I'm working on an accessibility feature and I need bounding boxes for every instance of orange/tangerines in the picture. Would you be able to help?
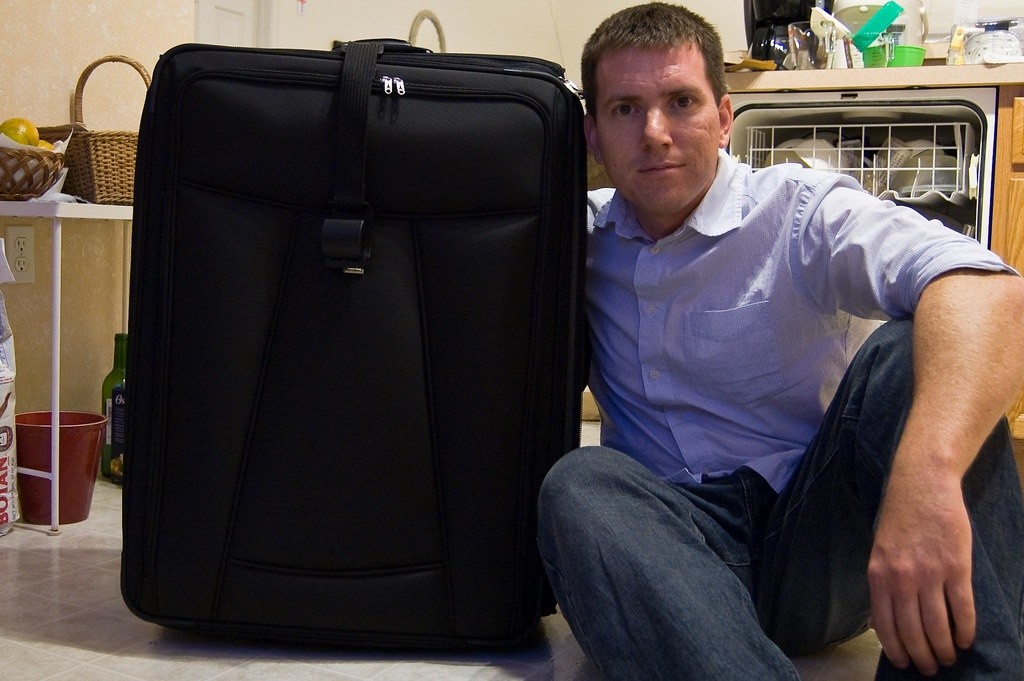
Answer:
[0,117,56,151]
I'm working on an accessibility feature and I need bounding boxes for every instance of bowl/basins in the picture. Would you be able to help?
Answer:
[764,132,958,198]
[862,43,927,68]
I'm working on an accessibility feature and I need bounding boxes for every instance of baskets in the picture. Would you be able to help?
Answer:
[43,54,152,206]
[0,147,66,202]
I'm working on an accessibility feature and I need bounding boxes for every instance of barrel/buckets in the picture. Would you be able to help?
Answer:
[15,410,109,527]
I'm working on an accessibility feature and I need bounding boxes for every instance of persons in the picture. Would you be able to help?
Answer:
[536,0,1023,681]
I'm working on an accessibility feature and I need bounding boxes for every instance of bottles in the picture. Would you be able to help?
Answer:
[100,331,127,479]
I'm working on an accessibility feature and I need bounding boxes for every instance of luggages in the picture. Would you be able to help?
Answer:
[119,30,591,655]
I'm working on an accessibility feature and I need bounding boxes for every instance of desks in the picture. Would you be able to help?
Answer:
[0,199,133,536]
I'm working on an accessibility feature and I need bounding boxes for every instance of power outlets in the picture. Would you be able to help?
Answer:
[6,226,34,283]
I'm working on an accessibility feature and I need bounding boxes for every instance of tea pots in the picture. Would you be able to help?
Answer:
[748,23,800,71]
